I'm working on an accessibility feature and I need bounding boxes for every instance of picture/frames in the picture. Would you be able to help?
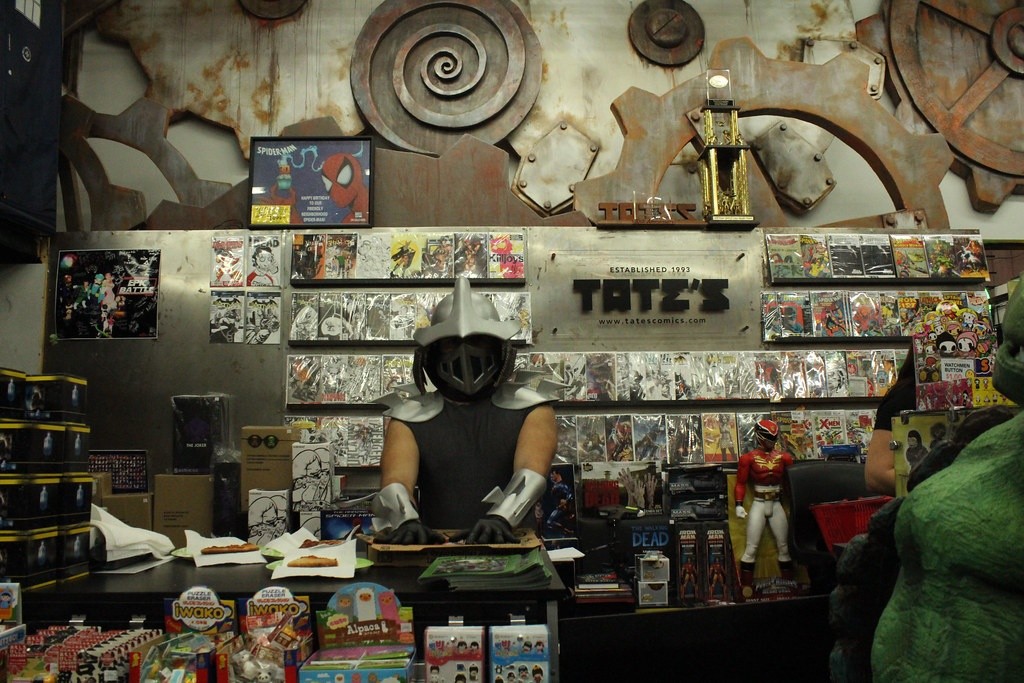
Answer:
[248,136,376,229]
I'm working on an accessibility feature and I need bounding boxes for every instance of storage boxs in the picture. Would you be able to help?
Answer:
[809,495,895,555]
[0,363,542,683]
[633,553,671,606]
[299,646,418,683]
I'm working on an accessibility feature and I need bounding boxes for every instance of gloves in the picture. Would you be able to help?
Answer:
[449,515,520,544]
[373,519,445,545]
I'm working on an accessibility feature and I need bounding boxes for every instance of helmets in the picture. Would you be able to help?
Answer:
[411,276,523,396]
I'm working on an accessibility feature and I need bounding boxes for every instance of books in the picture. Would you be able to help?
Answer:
[760,290,1020,412]
[54,249,162,341]
[285,347,909,593]
[208,289,533,344]
[765,234,991,282]
[209,231,527,287]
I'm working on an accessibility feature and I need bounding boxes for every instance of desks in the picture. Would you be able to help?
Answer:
[557,546,833,683]
[27,530,565,683]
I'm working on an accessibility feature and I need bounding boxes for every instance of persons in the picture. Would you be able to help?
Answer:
[710,558,728,596]
[864,343,916,492]
[380,292,558,544]
[682,557,697,597]
[734,420,794,580]
[62,273,116,335]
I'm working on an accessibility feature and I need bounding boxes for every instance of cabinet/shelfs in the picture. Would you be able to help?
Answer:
[0,0,65,265]
